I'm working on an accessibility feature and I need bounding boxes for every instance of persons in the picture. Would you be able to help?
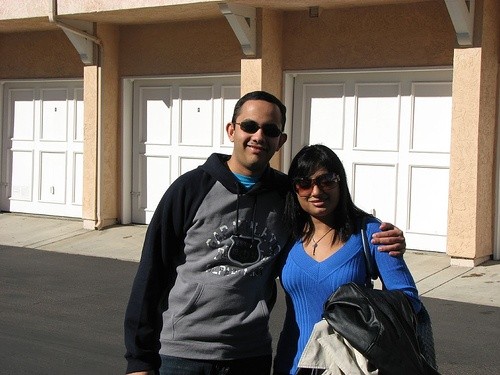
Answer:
[272,144,438,375]
[125,91,407,375]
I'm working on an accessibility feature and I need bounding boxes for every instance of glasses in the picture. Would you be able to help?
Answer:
[292,171,341,197]
[233,120,283,138]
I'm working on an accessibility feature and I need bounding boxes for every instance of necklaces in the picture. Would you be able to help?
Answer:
[311,228,333,255]
